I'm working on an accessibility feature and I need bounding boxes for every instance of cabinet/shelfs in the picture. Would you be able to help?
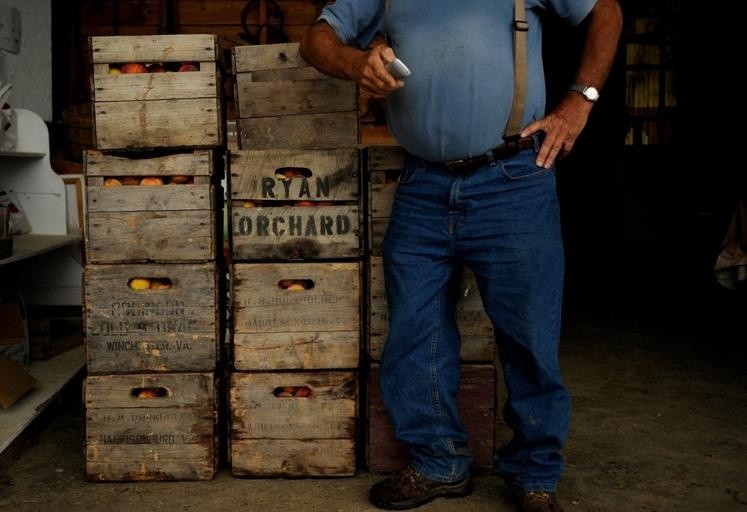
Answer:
[0,104,87,471]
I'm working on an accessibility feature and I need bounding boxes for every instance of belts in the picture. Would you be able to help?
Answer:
[409,129,546,176]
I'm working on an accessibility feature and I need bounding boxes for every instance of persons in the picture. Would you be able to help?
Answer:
[298,1,623,511]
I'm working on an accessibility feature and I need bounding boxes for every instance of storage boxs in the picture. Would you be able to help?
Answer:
[82,33,403,480]
[453,266,495,474]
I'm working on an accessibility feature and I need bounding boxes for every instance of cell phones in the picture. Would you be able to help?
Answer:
[385,58,411,80]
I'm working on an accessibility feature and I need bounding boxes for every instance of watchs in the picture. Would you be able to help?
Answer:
[571,84,601,104]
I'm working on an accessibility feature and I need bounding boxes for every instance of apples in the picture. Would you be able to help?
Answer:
[129,279,171,289]
[277,387,311,397]
[137,390,159,398]
[280,280,308,291]
[242,169,335,207]
[108,62,199,74]
[104,175,194,187]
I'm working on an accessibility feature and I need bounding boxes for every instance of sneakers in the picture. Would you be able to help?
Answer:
[367,463,475,511]
[502,482,564,512]
